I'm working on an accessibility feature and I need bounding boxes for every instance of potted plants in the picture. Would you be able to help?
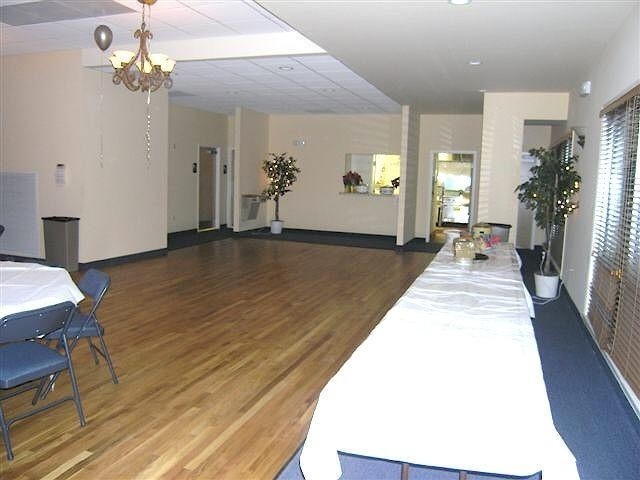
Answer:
[259,152,302,235]
[514,146,581,300]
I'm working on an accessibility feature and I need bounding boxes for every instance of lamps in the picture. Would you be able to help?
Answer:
[109,23,175,91]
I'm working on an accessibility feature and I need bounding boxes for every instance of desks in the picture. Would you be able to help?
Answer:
[0,259,71,318]
[315,238,580,480]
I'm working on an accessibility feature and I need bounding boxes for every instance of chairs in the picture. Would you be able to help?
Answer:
[40,268,119,400]
[1,301,85,461]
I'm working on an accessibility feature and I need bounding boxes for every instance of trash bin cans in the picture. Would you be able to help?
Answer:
[41,217,80,273]
[488,223,512,242]
